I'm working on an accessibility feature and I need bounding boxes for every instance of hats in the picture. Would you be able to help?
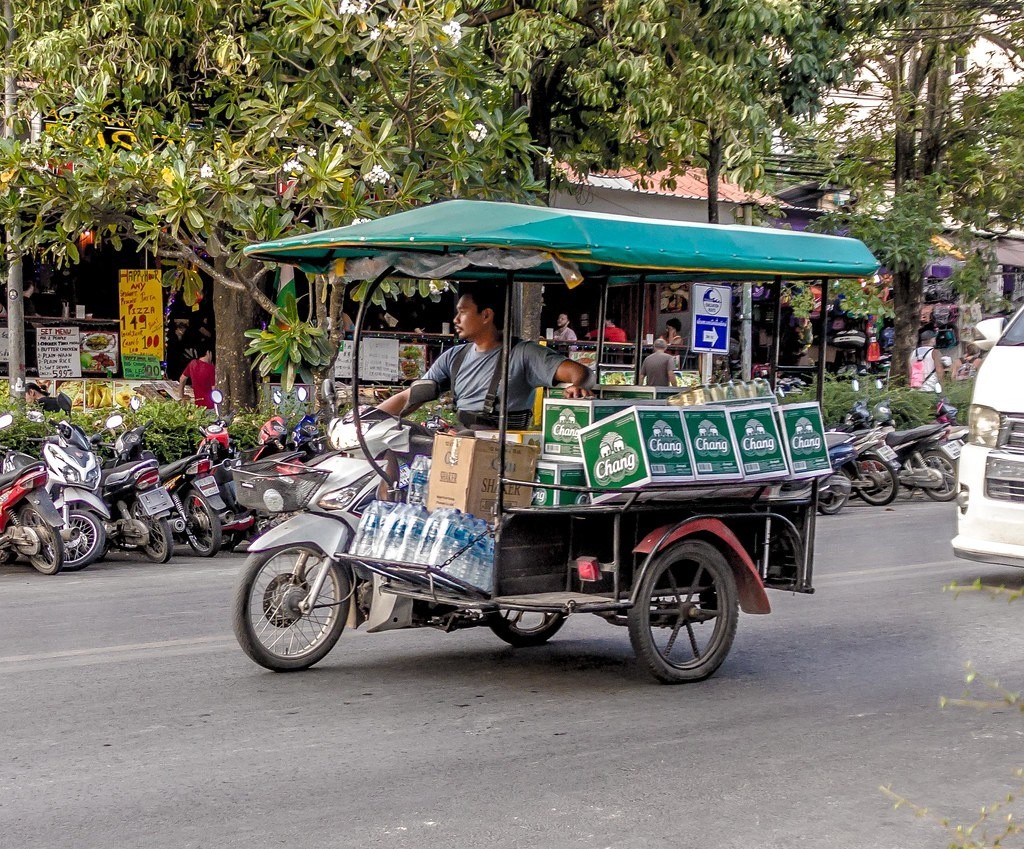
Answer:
[921,331,938,340]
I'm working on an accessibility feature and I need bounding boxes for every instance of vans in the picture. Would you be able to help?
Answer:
[950,303,1023,568]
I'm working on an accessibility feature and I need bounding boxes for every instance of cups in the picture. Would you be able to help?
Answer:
[63,307,69,317]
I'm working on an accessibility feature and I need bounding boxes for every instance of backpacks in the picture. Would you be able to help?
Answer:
[910,347,937,388]
[881,280,959,350]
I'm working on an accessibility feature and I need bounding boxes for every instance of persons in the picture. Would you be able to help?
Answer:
[638,337,678,387]
[50,246,88,317]
[167,296,216,412]
[553,311,578,341]
[908,330,948,393]
[366,303,426,332]
[23,281,45,329]
[951,343,986,382]
[584,312,627,343]
[373,284,597,440]
[25,382,68,416]
[658,317,685,345]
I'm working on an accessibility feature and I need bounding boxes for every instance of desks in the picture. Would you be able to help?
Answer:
[548,339,699,371]
[361,329,455,368]
[25,315,120,332]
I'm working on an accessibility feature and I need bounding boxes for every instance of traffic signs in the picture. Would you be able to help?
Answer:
[692,281,731,355]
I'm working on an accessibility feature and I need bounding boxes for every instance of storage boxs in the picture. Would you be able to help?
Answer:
[428,385,833,525]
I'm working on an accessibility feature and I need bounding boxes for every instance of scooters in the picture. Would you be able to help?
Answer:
[193,385,457,554]
[232,199,881,687]
[100,458,175,564]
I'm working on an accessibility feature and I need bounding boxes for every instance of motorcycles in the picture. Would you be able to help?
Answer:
[26,415,124,573]
[1,414,67,576]
[816,379,970,514]
[86,417,227,558]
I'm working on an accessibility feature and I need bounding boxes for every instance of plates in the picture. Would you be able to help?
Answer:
[82,333,116,353]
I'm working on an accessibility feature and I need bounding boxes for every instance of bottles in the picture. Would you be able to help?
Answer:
[348,498,495,594]
[406,454,433,508]
